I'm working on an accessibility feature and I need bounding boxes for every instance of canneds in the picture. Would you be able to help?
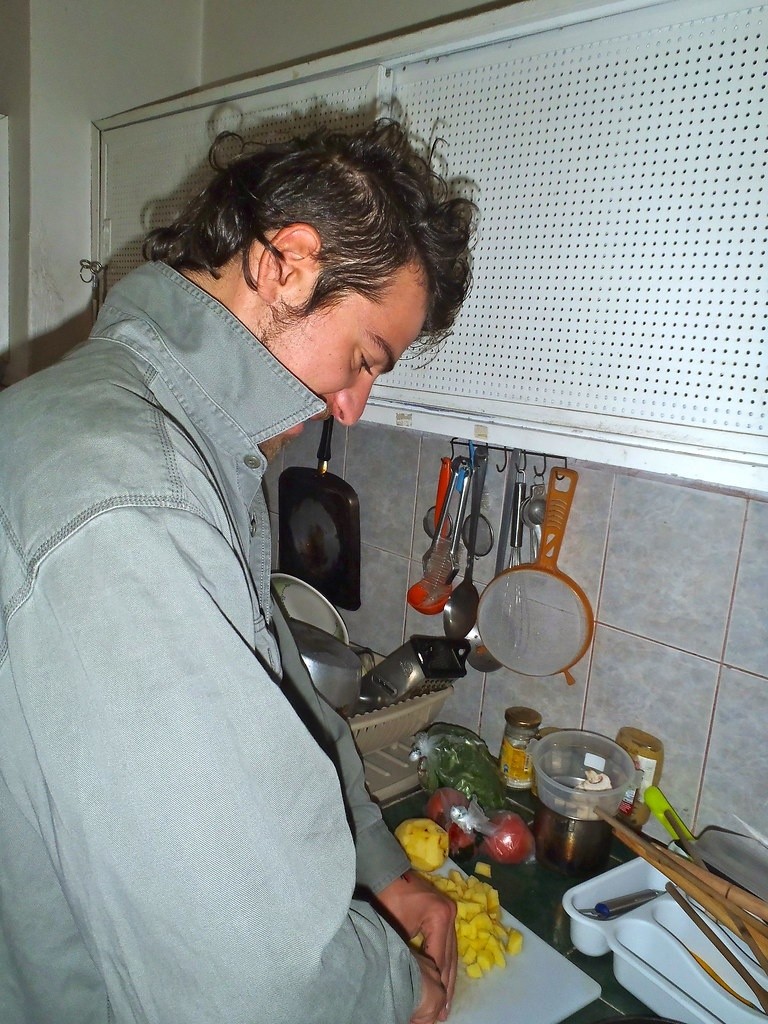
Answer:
[610,726,664,834]
[498,707,542,791]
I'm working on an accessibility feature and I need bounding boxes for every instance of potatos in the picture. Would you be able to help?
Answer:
[406,862,524,978]
[392,817,449,873]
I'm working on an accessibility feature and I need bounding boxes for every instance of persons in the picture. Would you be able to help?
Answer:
[1,110,492,1024]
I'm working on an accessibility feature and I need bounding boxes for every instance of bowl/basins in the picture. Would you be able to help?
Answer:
[526,732,636,820]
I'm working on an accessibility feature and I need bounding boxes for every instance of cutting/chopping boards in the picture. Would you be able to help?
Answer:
[426,859,602,1024]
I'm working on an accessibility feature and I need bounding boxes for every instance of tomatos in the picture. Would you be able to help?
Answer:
[476,815,536,864]
[428,788,474,847]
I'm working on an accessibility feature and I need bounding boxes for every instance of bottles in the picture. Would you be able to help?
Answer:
[616,728,664,835]
[497,707,543,791]
[530,725,563,799]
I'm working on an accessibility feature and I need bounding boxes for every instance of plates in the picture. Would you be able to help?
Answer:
[270,572,348,645]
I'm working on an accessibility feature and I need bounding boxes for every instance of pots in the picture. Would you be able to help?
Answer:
[277,416,362,611]
[476,465,594,686]
[531,800,613,876]
